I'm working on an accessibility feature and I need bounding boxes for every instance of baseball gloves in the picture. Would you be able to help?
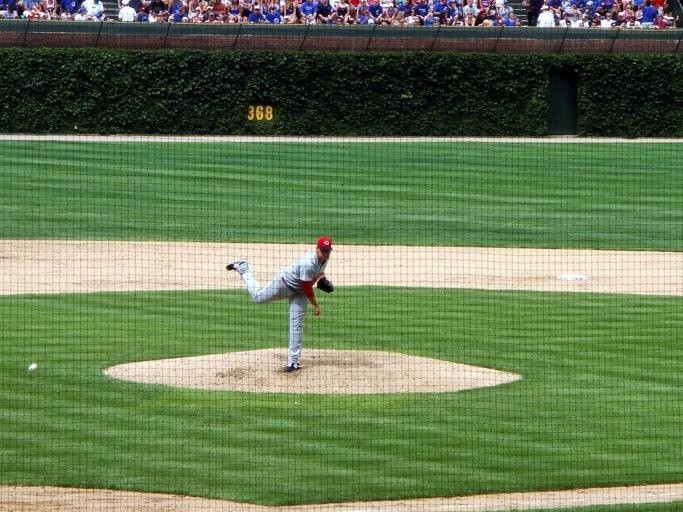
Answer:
[316,277,334,293]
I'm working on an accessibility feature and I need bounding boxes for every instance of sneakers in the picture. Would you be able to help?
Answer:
[288,362,302,371]
[225,257,248,271]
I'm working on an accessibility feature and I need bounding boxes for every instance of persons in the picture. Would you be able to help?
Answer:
[224,235,334,375]
[2,1,682,35]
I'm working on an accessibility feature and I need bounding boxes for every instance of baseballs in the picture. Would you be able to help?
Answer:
[28,363,39,373]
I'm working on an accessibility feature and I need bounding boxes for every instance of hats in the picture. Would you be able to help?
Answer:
[318,236,332,250]
